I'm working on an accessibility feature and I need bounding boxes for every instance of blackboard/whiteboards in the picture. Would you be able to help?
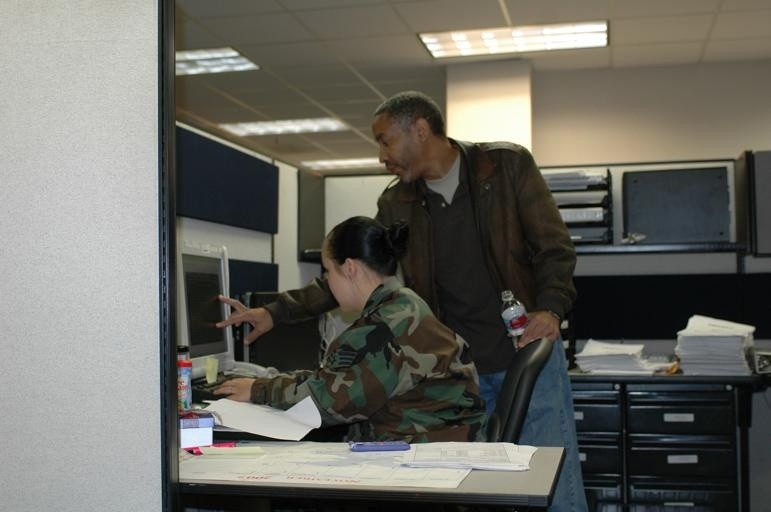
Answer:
[324,173,399,240]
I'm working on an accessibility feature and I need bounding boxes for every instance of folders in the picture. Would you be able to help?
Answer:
[747,345,770,374]
[542,170,614,245]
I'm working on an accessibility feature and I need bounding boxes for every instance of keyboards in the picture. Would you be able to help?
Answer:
[189,375,241,401]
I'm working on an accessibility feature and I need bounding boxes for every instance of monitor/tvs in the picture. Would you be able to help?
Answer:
[175,127,278,234]
[243,292,321,370]
[229,258,279,300]
[180,251,234,374]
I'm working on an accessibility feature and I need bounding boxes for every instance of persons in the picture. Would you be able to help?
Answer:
[212,216,487,442]
[216,92,589,511]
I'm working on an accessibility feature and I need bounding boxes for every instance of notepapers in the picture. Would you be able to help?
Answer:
[205,356,219,386]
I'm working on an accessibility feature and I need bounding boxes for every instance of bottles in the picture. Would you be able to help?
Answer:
[500,290,534,354]
[176,345,193,416]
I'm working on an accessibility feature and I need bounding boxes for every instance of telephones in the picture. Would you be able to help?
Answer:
[221,359,279,380]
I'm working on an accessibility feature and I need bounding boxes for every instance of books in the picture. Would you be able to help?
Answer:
[542,169,611,244]
[573,337,655,376]
[675,314,755,376]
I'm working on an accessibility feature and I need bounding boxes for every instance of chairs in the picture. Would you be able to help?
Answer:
[487,336,552,444]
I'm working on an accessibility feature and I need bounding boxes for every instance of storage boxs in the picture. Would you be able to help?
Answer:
[584,480,626,511]
[575,394,624,434]
[575,438,624,480]
[629,441,739,482]
[626,396,736,438]
[629,485,740,512]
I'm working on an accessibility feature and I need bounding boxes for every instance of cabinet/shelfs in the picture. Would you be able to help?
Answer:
[296,153,771,275]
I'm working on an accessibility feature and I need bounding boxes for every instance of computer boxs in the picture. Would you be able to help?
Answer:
[620,166,731,246]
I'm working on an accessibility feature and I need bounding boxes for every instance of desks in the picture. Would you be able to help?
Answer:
[565,336,770,511]
[180,433,567,511]
